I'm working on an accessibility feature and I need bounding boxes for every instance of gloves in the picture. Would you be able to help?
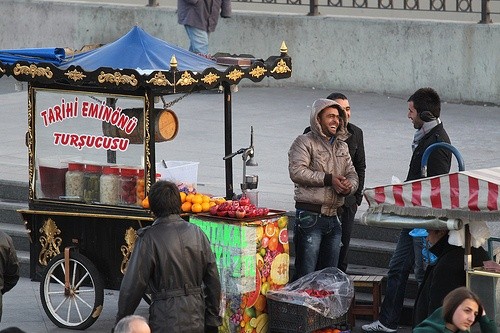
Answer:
[204,325,219,333]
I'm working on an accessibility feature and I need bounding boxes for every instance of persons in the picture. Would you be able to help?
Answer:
[361,87,453,333]
[412,286,500,333]
[177,0,233,56]
[0,229,20,325]
[114,315,152,333]
[288,100,360,283]
[108,180,221,333]
[412,215,495,333]
[301,93,365,274]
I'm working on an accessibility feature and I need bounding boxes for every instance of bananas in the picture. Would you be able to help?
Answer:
[254,313,269,333]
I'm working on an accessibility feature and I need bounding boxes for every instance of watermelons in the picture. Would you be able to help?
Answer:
[246,269,261,309]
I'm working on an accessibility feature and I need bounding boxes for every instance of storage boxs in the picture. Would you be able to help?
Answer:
[154,160,200,191]
[265,285,350,333]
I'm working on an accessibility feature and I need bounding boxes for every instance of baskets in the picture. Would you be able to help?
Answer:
[267,291,355,333]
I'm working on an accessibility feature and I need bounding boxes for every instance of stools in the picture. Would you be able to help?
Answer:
[349,276,384,326]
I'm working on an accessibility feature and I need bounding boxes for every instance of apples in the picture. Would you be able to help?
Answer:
[119,175,138,203]
[209,197,269,218]
[297,288,333,299]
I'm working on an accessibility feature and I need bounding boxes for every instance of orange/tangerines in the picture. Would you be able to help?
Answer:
[312,328,342,333]
[236,217,290,333]
[141,192,225,213]
[136,177,145,206]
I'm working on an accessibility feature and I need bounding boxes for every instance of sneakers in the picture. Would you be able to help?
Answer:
[361,320,398,333]
